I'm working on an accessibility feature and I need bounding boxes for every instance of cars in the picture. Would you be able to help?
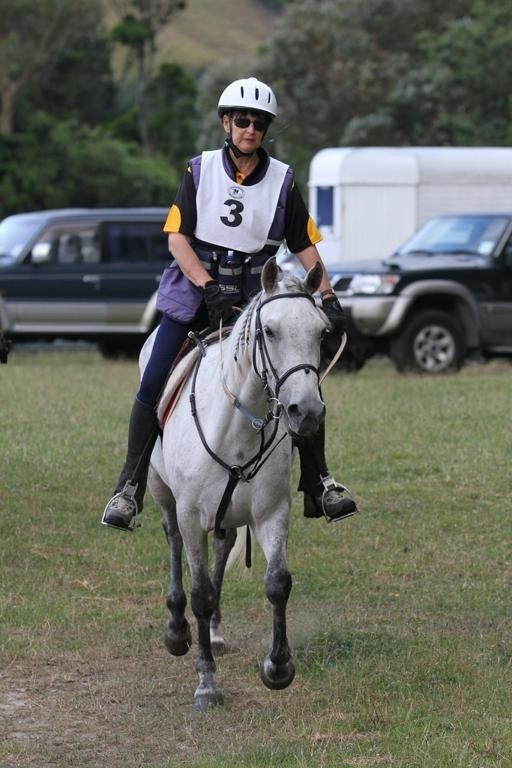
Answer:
[293,213,512,373]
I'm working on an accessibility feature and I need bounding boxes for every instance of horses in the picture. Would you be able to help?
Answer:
[138,256,328,713]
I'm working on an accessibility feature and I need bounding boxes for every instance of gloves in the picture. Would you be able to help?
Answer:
[320,298,347,333]
[195,279,234,322]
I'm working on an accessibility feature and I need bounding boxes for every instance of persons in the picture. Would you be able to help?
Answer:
[105,77,357,529]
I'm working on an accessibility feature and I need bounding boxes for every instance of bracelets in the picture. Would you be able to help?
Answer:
[320,289,334,301]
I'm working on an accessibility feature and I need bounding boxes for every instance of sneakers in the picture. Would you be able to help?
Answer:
[104,493,135,528]
[303,487,356,519]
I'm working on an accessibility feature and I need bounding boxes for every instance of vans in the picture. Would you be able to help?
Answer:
[1,207,173,363]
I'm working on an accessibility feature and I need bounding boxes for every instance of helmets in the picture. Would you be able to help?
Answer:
[216,76,278,118]
